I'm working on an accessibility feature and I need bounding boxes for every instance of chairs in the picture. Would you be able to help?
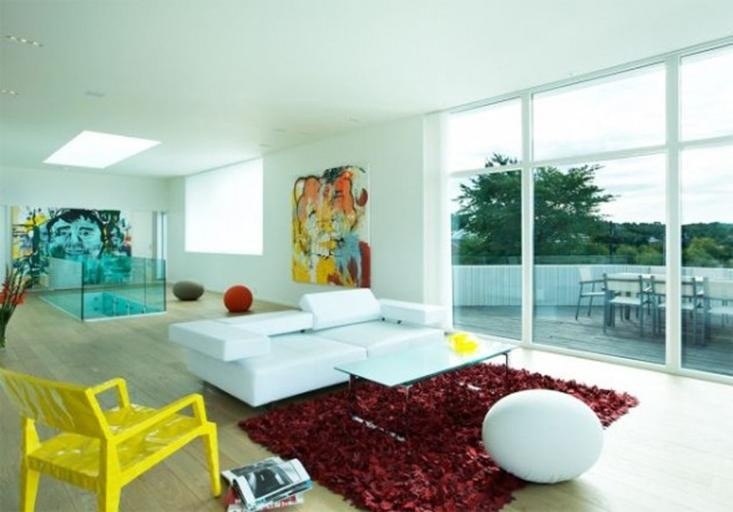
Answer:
[0,367,223,512]
[573,270,711,348]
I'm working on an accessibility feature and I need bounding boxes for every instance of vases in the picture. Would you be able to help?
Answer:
[0,250,40,319]
[1,304,17,348]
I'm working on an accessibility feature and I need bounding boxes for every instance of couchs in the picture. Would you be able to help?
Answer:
[167,298,452,408]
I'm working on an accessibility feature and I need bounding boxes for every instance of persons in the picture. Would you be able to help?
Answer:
[46,210,106,262]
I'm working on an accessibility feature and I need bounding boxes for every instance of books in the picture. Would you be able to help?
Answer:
[220,455,313,512]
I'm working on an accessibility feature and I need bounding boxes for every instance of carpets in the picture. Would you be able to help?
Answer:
[237,361,638,512]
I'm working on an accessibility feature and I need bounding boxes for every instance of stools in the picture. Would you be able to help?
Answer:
[481,389,608,486]
[172,281,204,302]
[224,285,252,312]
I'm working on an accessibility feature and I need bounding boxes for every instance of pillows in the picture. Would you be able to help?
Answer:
[299,287,384,331]
